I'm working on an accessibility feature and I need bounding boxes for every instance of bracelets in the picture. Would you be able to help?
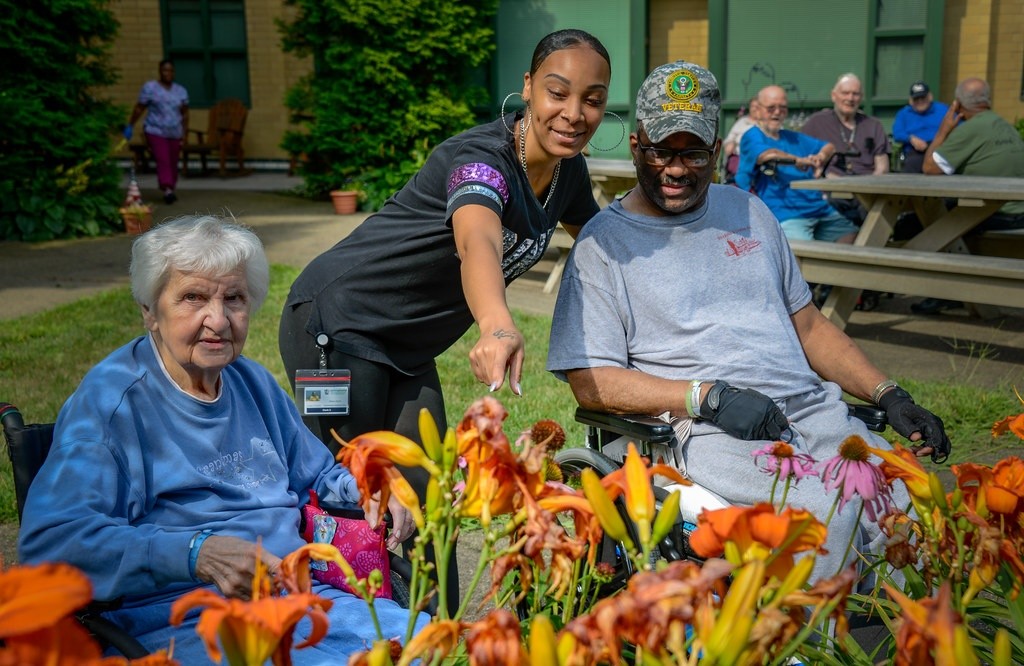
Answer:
[685,379,702,419]
[189,532,213,579]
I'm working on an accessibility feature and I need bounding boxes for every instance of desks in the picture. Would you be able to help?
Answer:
[788,175,1024,351]
[540,157,638,294]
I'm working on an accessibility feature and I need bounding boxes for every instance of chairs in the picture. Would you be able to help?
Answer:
[182,97,248,176]
[128,104,192,172]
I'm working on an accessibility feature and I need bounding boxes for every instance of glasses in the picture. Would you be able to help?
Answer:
[637,134,718,168]
[760,103,788,113]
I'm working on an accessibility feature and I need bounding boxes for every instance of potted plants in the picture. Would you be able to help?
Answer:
[118,204,155,234]
[329,181,360,213]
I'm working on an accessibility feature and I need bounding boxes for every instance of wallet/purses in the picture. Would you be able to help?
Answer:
[303,504,392,600]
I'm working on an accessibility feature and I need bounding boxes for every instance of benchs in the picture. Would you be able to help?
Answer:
[788,238,1024,310]
[547,228,576,250]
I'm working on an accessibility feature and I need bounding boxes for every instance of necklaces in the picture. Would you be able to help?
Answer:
[520,119,560,209]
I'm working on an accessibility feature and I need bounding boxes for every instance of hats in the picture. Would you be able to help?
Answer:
[910,82,928,100]
[635,60,721,147]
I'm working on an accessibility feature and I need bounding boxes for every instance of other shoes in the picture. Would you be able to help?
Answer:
[164,193,177,205]
[818,289,880,312]
[911,298,965,313]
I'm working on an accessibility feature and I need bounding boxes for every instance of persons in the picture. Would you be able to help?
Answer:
[310,391,320,401]
[544,59,952,666]
[277,28,612,620]
[17,206,435,666]
[130,59,189,204]
[724,71,1024,316]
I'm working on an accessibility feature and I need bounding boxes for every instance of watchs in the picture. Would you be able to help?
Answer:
[872,379,898,405]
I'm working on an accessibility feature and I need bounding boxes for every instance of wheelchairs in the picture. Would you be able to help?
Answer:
[0,399,448,666]
[510,401,895,666]
[725,147,880,313]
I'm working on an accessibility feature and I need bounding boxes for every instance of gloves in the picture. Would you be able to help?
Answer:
[700,380,789,442]
[124,125,133,141]
